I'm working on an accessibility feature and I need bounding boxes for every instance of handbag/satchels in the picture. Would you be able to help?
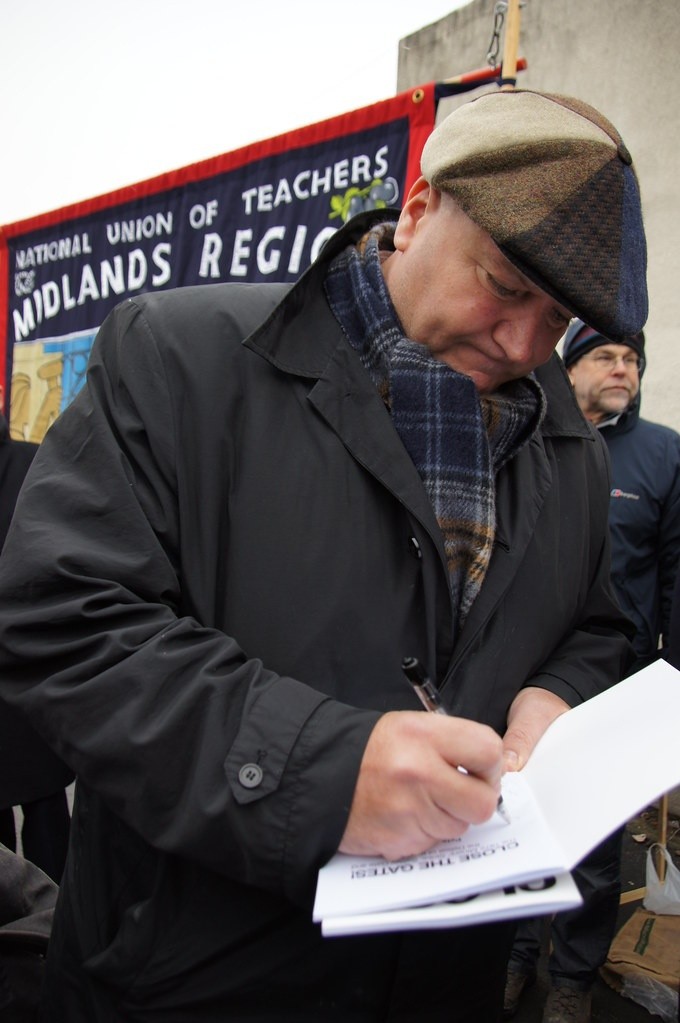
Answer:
[643,843,680,915]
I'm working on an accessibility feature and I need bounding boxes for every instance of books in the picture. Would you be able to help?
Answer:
[313,658,680,937]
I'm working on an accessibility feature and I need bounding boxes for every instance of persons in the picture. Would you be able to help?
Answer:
[0,414,77,885]
[504,321,680,1023]
[0,90,650,1023]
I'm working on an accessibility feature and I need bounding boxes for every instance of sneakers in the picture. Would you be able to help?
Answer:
[503,967,537,1018]
[542,986,591,1023]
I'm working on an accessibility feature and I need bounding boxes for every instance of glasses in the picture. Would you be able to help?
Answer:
[581,354,644,371]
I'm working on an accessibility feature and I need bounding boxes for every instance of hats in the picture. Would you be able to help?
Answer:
[562,318,646,379]
[420,89,649,344]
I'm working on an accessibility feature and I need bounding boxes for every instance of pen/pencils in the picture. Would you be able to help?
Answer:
[403,657,512,826]
